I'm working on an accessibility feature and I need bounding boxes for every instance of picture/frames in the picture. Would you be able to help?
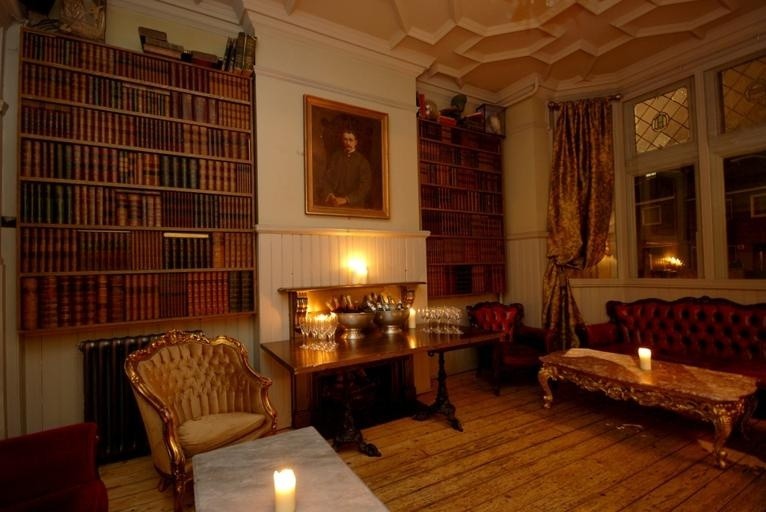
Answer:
[303,94,391,219]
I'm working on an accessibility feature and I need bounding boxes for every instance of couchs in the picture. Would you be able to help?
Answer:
[124,330,278,512]
[467,303,556,395]
[576,296,766,390]
[0,422,109,512]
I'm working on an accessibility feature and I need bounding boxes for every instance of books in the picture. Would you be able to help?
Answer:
[23,31,250,102]
[420,139,500,172]
[21,138,252,193]
[21,271,254,331]
[138,25,256,76]
[419,120,501,153]
[20,183,252,229]
[419,162,501,193]
[20,226,253,272]
[416,90,483,131]
[427,237,504,264]
[22,100,252,162]
[428,264,505,296]
[421,185,502,214]
[422,210,503,238]
[23,62,250,131]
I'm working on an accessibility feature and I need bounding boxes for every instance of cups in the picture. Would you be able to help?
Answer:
[638,347,652,370]
[418,307,460,335]
[298,312,339,353]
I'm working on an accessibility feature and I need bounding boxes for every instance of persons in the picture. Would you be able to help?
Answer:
[322,129,372,208]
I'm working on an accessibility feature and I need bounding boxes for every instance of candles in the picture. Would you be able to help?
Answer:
[639,346,651,370]
[347,258,370,286]
[408,307,417,328]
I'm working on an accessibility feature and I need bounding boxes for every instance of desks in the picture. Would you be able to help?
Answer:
[260,322,506,457]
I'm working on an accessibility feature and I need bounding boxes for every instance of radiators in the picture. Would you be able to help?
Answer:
[78,333,207,463]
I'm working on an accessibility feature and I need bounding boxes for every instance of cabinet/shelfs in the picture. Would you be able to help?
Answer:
[22,32,254,330]
[418,118,508,299]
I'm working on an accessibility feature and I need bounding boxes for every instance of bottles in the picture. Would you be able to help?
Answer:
[341,294,348,313]
[332,297,341,309]
[325,302,335,313]
[361,291,403,312]
[346,295,355,313]
